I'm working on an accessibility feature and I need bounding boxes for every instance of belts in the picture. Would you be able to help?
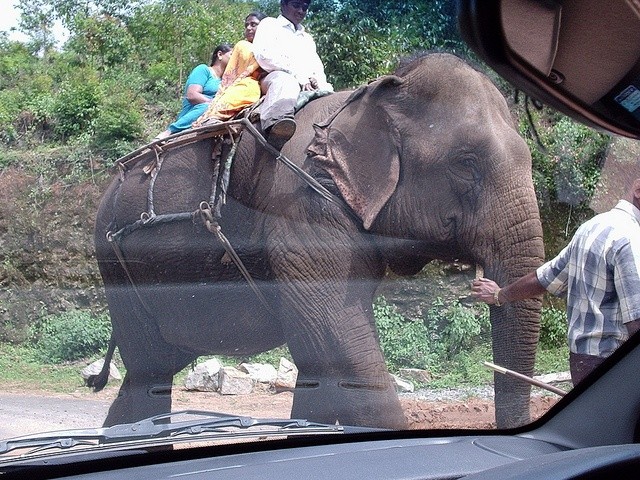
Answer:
[568,350,608,363]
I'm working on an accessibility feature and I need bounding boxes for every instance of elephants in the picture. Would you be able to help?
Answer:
[87,51,546,451]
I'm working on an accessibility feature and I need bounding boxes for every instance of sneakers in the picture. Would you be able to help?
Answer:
[266,117,298,153]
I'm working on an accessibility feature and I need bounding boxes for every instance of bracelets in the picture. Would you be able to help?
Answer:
[494,289,502,307]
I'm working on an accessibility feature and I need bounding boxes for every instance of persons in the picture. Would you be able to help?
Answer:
[153,44,234,144]
[252,0,332,151]
[191,13,268,127]
[467,176,639,386]
